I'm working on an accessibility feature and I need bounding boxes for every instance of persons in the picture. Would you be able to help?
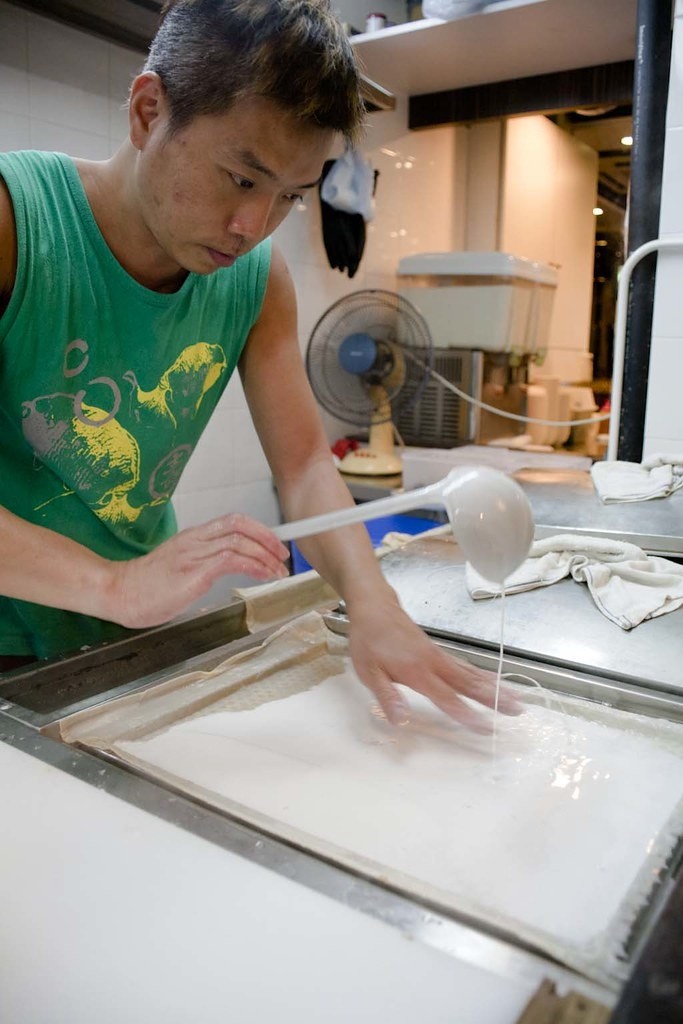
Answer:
[0,0,528,738]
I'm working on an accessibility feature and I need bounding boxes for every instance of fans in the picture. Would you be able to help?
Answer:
[306,285,434,476]
